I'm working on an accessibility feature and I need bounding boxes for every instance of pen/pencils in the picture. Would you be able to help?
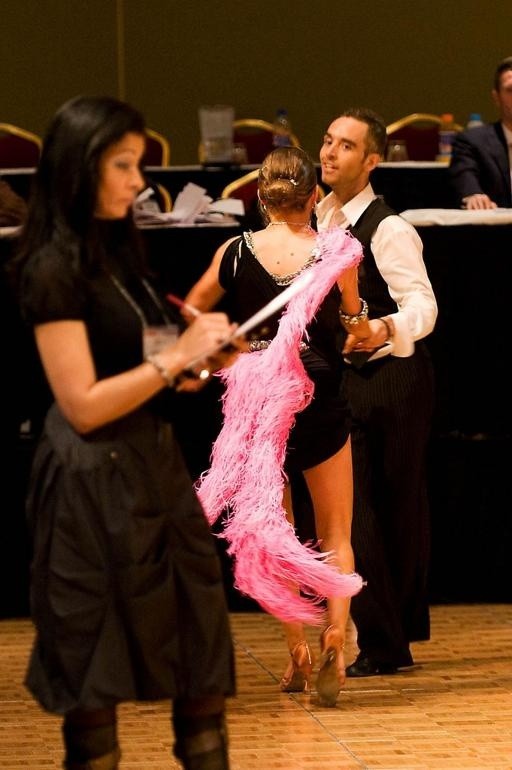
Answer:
[167,295,200,318]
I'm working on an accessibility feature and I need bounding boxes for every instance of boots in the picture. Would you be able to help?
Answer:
[62,720,119,770]
[173,712,229,770]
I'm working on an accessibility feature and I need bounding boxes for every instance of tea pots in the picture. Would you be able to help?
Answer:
[197,103,236,171]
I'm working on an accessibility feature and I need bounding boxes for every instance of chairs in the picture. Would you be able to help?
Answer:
[0,121,45,167]
[371,113,464,162]
[134,126,172,167]
[196,117,302,163]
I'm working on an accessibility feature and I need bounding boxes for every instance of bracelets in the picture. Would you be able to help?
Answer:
[377,318,391,341]
[338,298,369,325]
[145,354,176,389]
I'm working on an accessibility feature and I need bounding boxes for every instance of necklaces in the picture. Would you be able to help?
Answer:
[265,222,306,229]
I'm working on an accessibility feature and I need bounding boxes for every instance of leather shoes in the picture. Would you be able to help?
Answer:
[345,649,414,677]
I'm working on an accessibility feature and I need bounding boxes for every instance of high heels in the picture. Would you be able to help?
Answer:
[317,624,345,699]
[279,640,314,693]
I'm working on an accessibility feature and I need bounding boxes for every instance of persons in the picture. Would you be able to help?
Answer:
[178,146,373,708]
[308,104,439,678]
[449,55,512,210]
[1,92,241,770]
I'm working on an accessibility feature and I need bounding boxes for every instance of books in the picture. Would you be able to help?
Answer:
[133,182,245,227]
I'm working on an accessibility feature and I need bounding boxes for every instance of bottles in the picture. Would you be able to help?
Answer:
[271,107,292,147]
[466,112,485,130]
[438,112,458,165]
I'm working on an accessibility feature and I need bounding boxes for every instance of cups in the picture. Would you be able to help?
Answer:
[230,143,247,165]
[385,139,409,163]
